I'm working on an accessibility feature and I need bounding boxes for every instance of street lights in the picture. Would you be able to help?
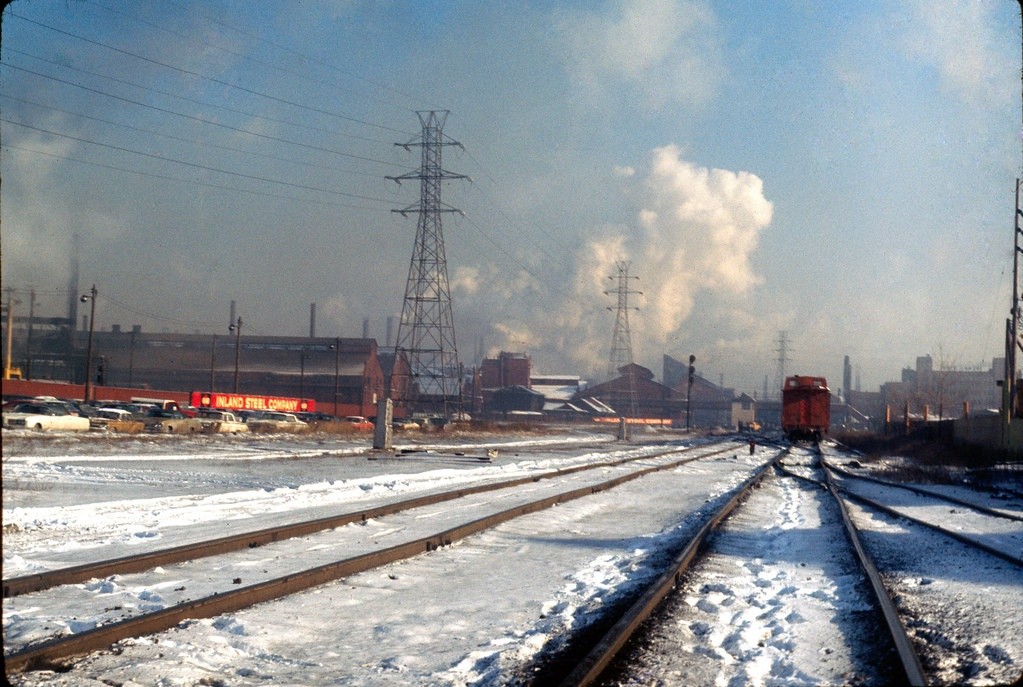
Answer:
[228,315,242,394]
[80,283,97,404]
[330,337,340,416]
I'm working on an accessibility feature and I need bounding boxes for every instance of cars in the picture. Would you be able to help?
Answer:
[2,395,375,434]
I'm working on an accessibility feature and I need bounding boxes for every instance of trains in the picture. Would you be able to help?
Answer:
[781,375,831,444]
